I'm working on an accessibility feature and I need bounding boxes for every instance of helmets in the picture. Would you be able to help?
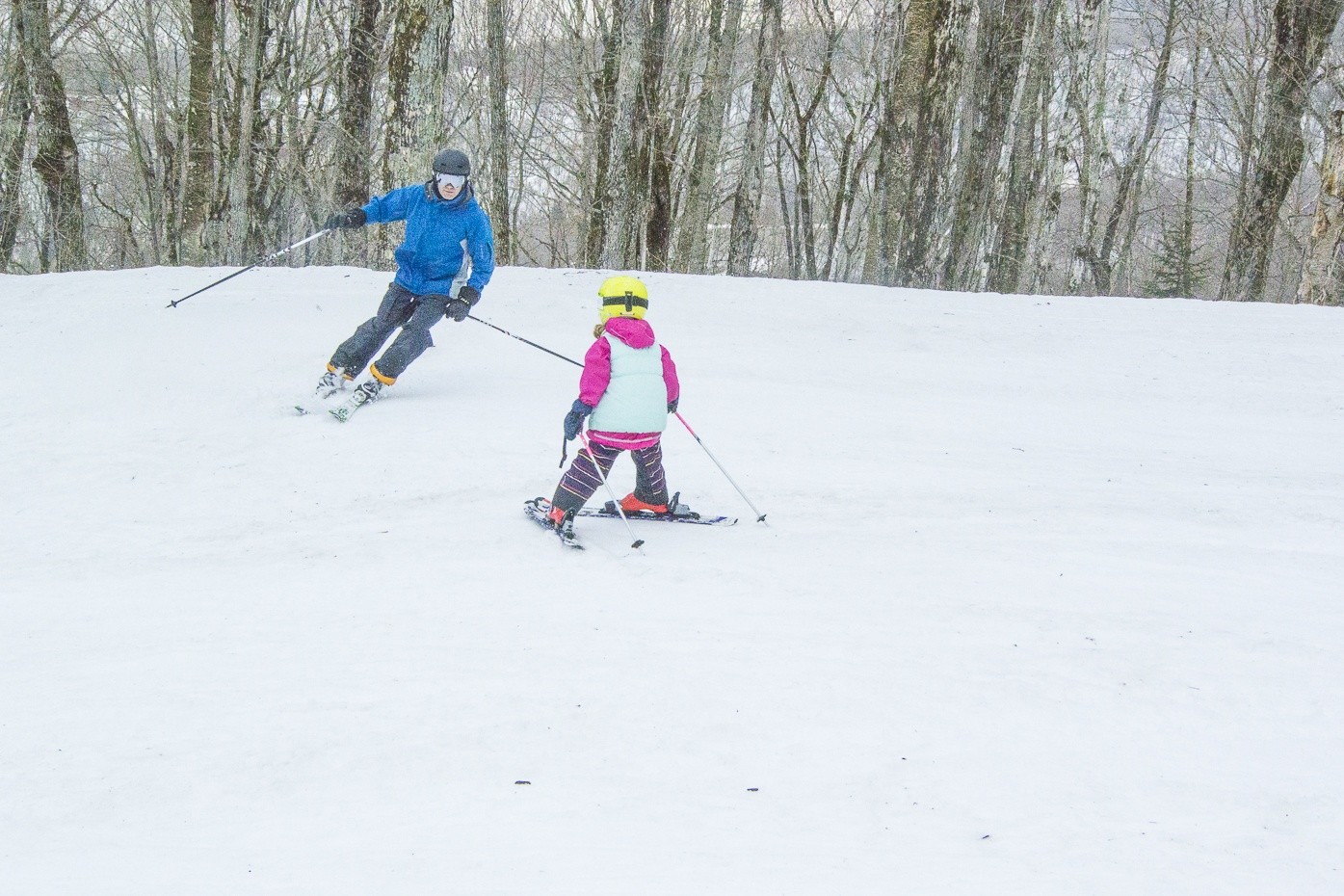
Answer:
[432,147,470,177]
[596,276,649,324]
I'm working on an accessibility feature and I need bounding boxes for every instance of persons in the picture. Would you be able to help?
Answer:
[546,275,682,529]
[318,146,497,404]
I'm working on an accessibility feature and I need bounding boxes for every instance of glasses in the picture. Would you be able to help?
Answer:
[435,172,466,188]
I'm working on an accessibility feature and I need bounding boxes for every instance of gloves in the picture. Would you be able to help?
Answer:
[563,399,592,442]
[442,285,478,322]
[323,208,368,231]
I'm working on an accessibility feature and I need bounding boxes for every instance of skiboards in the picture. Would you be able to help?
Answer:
[521,496,766,554]
[293,368,375,422]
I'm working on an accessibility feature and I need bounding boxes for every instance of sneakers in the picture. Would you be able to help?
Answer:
[316,370,346,392]
[352,377,383,404]
[545,503,566,526]
[618,493,668,515]
